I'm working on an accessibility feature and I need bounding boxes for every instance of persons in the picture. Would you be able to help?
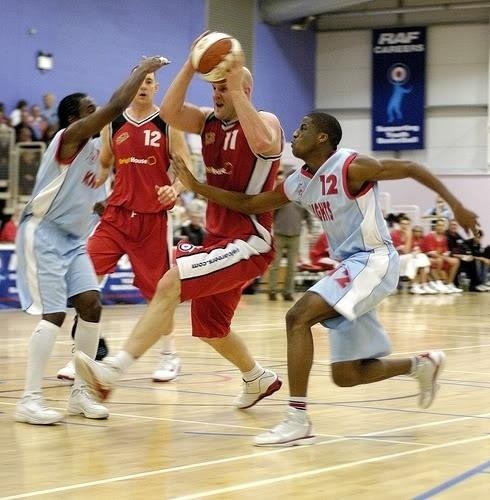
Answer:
[169,112,483,446]
[57,64,193,384]
[68,28,286,410]
[10,54,172,426]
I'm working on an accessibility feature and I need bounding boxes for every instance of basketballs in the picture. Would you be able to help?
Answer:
[191,32,241,83]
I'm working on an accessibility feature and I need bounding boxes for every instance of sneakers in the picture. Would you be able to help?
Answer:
[67,383,109,419]
[237,368,281,411]
[15,393,62,424]
[253,406,315,447]
[413,349,445,410]
[74,351,118,402]
[284,293,294,301]
[269,291,277,301]
[152,353,181,382]
[56,343,75,383]
[70,317,106,359]
[409,280,490,294]
